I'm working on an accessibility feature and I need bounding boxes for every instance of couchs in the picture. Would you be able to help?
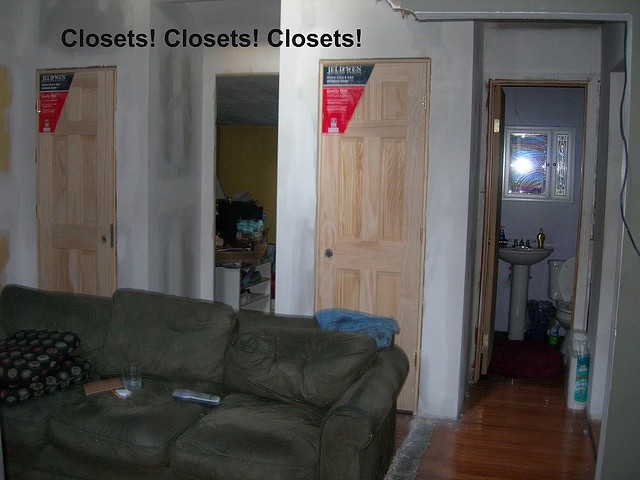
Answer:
[0,285,409,480]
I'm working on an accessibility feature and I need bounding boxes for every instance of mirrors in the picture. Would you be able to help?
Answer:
[503,125,551,204]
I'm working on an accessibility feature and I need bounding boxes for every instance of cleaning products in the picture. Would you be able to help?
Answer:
[549,319,558,346]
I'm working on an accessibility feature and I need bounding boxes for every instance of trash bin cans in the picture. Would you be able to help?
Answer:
[526,299,557,342]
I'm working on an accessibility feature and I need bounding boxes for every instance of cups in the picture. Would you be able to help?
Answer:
[122,362,143,391]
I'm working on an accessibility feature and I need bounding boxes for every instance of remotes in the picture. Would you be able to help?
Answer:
[172,388,220,405]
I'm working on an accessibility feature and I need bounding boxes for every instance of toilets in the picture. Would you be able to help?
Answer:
[548,257,576,330]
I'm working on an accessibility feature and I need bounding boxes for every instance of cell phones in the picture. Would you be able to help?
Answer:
[116,388,131,399]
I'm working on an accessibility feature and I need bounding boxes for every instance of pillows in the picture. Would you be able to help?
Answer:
[0,328,81,384]
[0,356,91,405]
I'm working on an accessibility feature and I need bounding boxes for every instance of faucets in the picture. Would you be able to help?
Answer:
[520,238,524,246]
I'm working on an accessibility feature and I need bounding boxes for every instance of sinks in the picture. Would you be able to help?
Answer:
[499,247,553,265]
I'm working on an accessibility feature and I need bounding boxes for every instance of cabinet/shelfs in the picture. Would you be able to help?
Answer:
[214,259,271,315]
[552,126,573,202]
